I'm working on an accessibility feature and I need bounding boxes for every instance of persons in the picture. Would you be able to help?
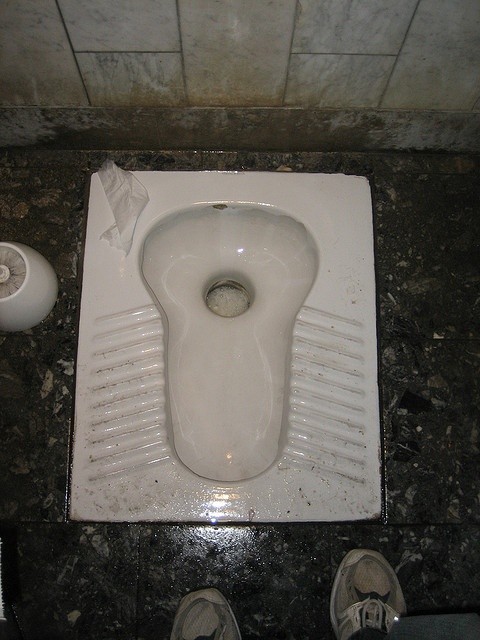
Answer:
[167,546,479,640]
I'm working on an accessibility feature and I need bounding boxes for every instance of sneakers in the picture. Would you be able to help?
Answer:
[170,588,242,640]
[329,549,407,640]
